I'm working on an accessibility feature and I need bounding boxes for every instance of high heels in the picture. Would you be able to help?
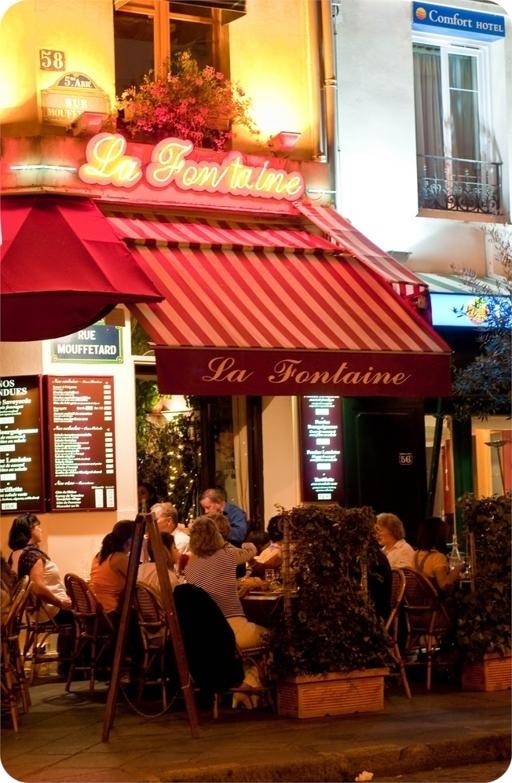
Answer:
[231,691,264,712]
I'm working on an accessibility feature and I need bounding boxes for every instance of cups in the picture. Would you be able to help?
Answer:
[265,568,275,587]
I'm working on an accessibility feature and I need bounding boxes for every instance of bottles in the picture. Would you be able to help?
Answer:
[450,534,461,572]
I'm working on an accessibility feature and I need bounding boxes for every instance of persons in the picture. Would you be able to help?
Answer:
[7,512,92,681]
[355,546,391,621]
[0,552,18,621]
[412,518,468,687]
[376,514,414,570]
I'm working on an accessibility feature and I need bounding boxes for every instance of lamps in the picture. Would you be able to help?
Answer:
[68,110,110,137]
[267,130,305,158]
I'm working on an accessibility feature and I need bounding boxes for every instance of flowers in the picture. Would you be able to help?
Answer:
[122,47,262,154]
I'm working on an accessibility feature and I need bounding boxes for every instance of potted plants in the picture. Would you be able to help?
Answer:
[271,498,397,717]
[456,487,511,694]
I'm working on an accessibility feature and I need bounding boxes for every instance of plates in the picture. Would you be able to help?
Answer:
[248,591,282,596]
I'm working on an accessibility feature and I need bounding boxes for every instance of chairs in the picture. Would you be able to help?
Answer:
[379,566,455,699]
[1,574,270,732]
